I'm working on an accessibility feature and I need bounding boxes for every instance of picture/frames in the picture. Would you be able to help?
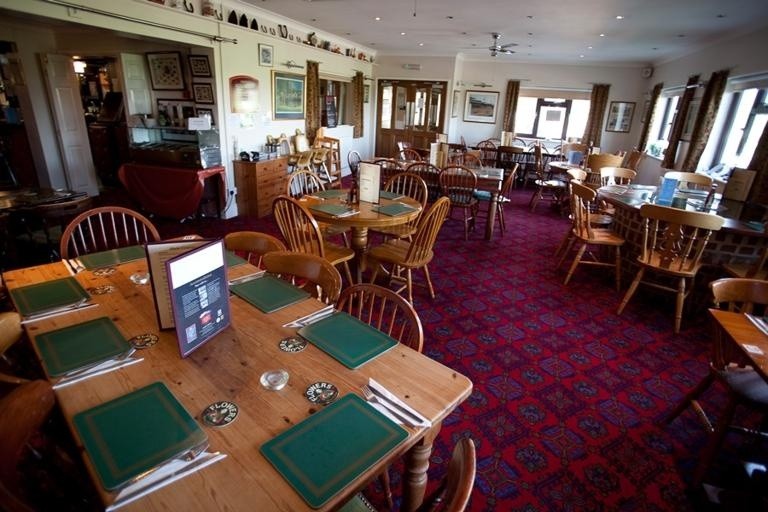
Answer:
[188,54,212,77]
[462,89,500,124]
[258,43,275,67]
[191,82,214,105]
[144,51,187,91]
[157,98,194,126]
[196,108,214,126]
[604,101,636,134]
[270,69,307,121]
[678,97,703,142]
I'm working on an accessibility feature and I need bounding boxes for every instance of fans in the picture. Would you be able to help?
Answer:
[458,31,520,57]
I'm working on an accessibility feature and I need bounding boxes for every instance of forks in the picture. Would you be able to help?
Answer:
[23,297,86,318]
[64,341,137,385]
[360,384,415,430]
[299,311,336,329]
[129,442,209,486]
[72,257,85,272]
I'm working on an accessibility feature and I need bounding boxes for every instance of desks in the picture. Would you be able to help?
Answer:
[1,231,476,511]
[8,186,93,261]
[116,163,226,219]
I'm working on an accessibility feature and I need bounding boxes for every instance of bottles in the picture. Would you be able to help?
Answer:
[269,143,272,152]
[704,184,718,210]
[265,144,269,152]
[350,175,359,205]
[276,143,281,156]
[271,142,276,152]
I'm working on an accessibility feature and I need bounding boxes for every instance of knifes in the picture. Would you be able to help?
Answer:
[19,303,99,328]
[105,450,219,511]
[64,257,77,273]
[287,308,333,328]
[52,356,144,398]
[367,385,423,423]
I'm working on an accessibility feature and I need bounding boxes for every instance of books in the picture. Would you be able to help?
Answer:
[500,131,512,147]
[354,161,381,207]
[143,239,232,357]
[435,133,448,145]
[430,142,448,169]
[722,167,757,204]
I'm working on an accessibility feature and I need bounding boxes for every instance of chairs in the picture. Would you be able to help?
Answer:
[58,205,162,261]
[0,310,37,386]
[0,378,57,512]
